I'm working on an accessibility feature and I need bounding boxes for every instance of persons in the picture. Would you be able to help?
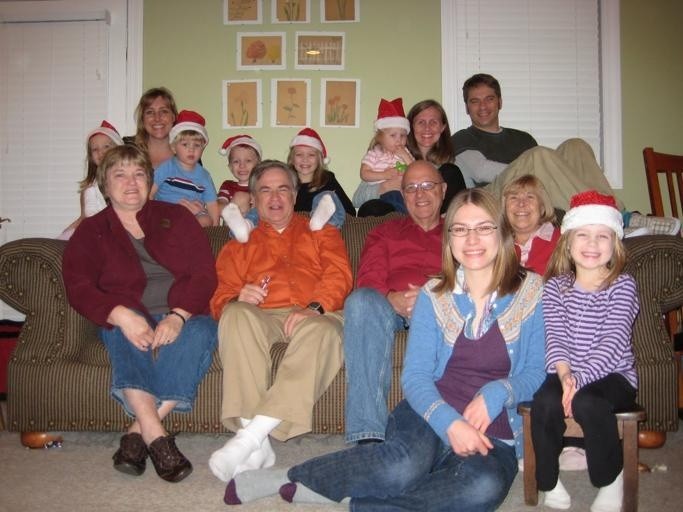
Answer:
[222,187,548,512]
[495,170,568,277]
[213,129,263,209]
[352,97,416,216]
[446,72,682,239]
[360,92,467,223]
[60,144,220,485]
[150,108,221,230]
[339,159,461,449]
[529,191,643,512]
[66,121,129,230]
[120,83,184,171]
[223,128,357,245]
[208,159,354,483]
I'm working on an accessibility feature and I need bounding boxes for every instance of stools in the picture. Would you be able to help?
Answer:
[516,401,646,512]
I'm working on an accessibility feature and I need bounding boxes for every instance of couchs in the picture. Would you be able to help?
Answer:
[0,213,683,449]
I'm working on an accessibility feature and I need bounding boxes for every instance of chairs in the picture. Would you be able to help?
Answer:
[643,146,683,350]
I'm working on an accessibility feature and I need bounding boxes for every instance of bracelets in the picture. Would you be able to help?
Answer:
[167,310,186,324]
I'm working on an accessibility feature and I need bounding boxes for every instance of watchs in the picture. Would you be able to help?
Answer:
[308,301,325,314]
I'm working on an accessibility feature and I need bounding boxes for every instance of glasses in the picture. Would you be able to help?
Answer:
[448,224,497,237]
[402,182,442,193]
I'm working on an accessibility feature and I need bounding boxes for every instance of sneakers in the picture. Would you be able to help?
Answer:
[148,434,193,482]
[624,213,680,239]
[113,431,148,476]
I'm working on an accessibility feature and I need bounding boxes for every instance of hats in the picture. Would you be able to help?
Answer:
[373,98,411,136]
[560,191,624,240]
[88,120,125,146]
[289,127,330,164]
[220,135,263,160]
[169,110,209,145]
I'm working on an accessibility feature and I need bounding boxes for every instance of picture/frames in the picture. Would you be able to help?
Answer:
[222,80,262,129]
[270,0,311,24]
[234,30,285,70]
[223,0,262,25]
[272,79,311,129]
[294,30,345,70]
[321,0,359,24]
[319,78,359,128]
